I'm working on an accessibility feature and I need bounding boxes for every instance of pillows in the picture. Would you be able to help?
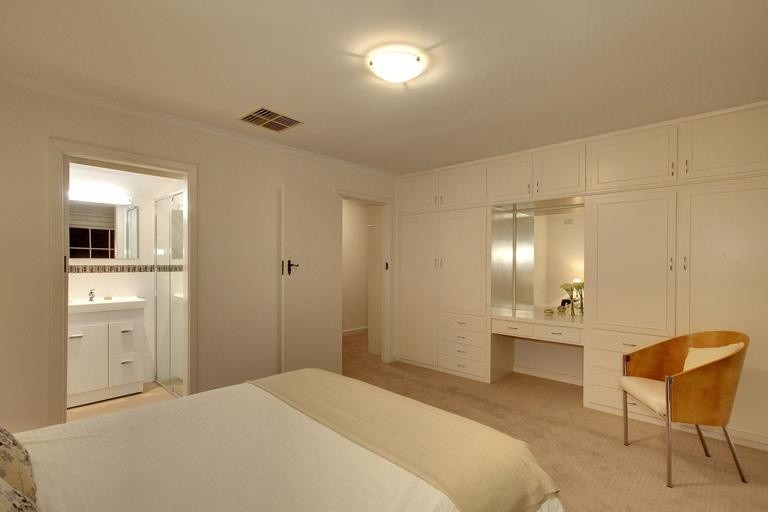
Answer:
[0,425,39,512]
[681,342,744,374]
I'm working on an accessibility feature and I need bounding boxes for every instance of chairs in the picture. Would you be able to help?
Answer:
[621,330,751,488]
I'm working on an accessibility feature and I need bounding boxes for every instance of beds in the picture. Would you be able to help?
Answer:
[3,366,548,512]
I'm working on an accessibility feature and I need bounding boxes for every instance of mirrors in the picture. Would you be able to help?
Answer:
[68,200,153,265]
[511,207,584,312]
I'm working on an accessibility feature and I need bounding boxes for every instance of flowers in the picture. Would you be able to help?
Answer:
[560,282,576,317]
[571,279,584,310]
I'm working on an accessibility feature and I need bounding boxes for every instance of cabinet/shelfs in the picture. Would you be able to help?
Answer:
[398,163,483,209]
[109,321,144,388]
[492,319,584,346]
[398,214,437,367]
[486,144,586,200]
[588,189,672,425]
[680,189,768,438]
[67,324,108,395]
[585,104,768,186]
[439,210,486,382]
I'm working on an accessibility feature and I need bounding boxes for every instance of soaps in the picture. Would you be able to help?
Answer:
[104,294,112,301]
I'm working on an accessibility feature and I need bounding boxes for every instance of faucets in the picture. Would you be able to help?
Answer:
[87,288,96,301]
